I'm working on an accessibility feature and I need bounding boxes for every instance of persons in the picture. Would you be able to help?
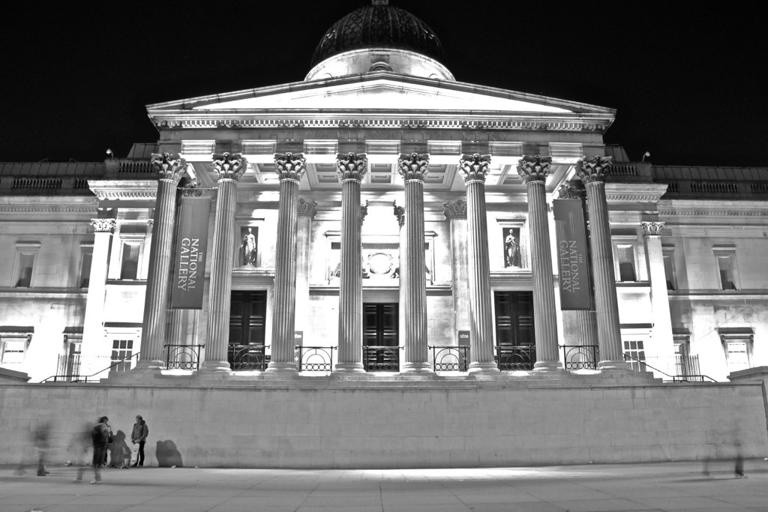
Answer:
[132,416,148,468]
[91,417,113,469]
